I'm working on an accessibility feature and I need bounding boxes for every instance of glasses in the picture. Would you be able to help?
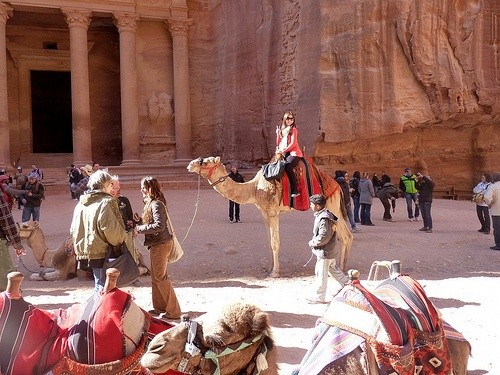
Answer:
[286,117,294,120]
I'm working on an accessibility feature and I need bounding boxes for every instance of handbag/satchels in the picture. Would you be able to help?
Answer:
[167,235,184,263]
[102,237,140,289]
[383,183,397,189]
[473,192,484,204]
[262,155,286,180]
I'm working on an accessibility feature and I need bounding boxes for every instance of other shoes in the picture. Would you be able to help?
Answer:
[148,309,166,316]
[489,245,500,250]
[230,220,233,223]
[477,229,484,232]
[292,192,300,197]
[162,315,182,322]
[350,217,432,233]
[236,220,242,223]
[484,230,490,234]
[308,298,324,304]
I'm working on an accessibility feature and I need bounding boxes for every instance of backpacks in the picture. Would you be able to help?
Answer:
[69,169,77,178]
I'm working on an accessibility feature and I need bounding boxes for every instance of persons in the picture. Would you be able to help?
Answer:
[372,172,379,197]
[381,171,391,188]
[484,172,500,250]
[0,187,26,293]
[333,170,361,232]
[0,164,45,229]
[473,174,493,234]
[68,162,99,200]
[110,175,141,287]
[358,171,375,226]
[308,194,350,304]
[70,169,129,293]
[399,168,419,221]
[414,171,435,233]
[228,164,244,223]
[349,171,361,223]
[378,185,405,222]
[276,110,304,197]
[127,176,182,322]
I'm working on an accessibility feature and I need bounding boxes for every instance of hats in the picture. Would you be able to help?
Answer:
[80,164,93,177]
[28,172,37,178]
[0,174,8,181]
[18,167,22,170]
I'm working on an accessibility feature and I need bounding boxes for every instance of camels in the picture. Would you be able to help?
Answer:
[18,220,152,287]
[141,274,470,375]
[187,156,354,278]
[0,287,175,375]
